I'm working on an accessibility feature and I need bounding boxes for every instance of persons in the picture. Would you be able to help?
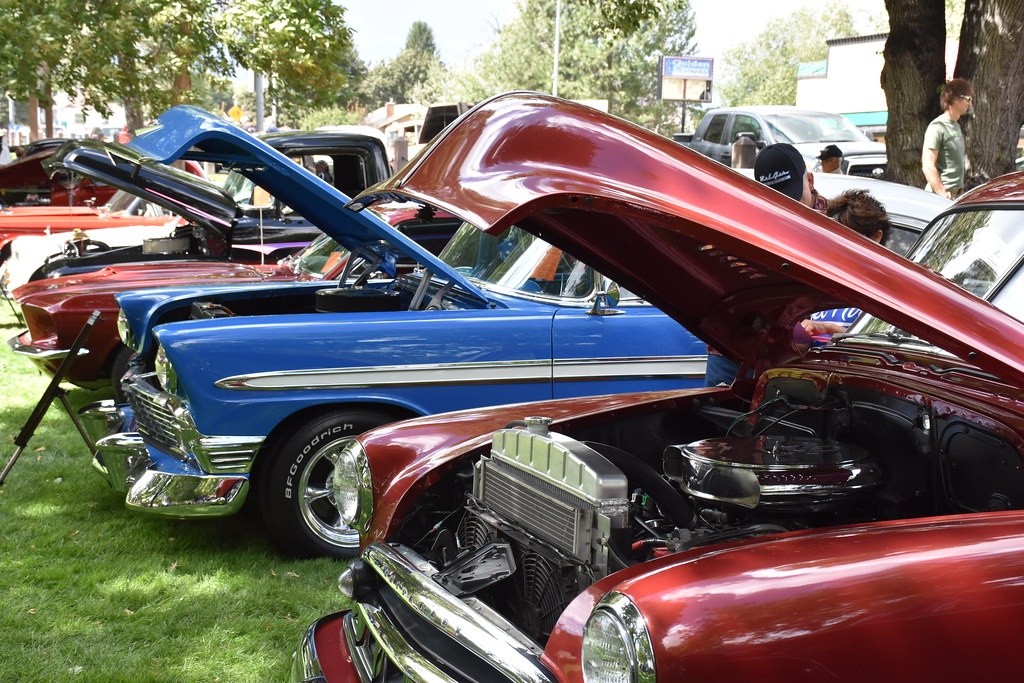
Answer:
[816,144,843,174]
[315,160,333,184]
[921,78,974,201]
[801,188,891,340]
[92,127,106,141]
[702,143,829,388]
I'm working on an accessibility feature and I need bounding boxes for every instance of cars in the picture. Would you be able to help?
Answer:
[115,101,954,563]
[730,165,956,259]
[8,178,620,399]
[26,129,394,290]
[683,107,891,189]
[286,90,1024,683]
[1,135,219,300]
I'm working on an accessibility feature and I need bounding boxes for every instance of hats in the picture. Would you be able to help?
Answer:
[816,144,842,161]
[754,143,806,201]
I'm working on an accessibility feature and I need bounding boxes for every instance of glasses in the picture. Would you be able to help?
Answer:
[960,95,972,102]
[820,159,832,165]
